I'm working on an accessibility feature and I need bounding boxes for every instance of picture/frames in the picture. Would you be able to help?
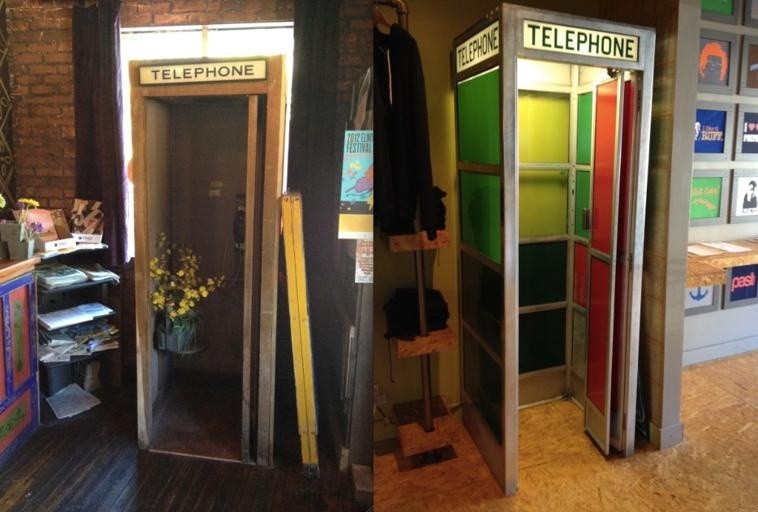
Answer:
[682,0,757,317]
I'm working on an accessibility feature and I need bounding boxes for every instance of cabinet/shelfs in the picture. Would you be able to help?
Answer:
[36,244,114,429]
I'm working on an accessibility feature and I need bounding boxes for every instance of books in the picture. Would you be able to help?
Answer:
[11,196,122,420]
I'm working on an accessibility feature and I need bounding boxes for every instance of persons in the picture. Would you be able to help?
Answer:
[741,180,756,208]
